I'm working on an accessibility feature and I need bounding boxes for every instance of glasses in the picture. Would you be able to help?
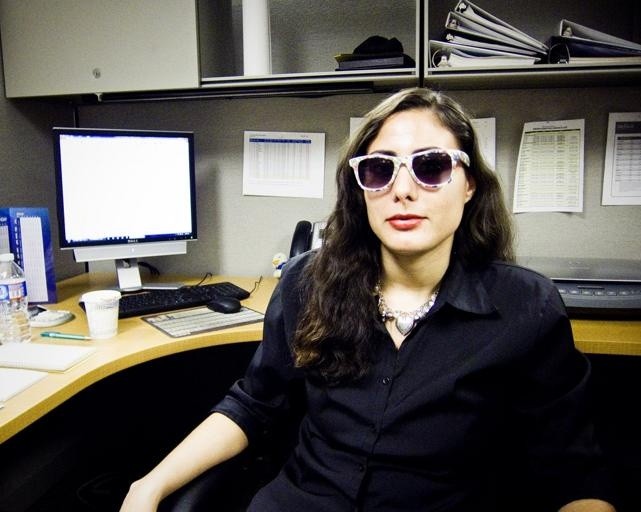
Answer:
[347,148,470,194]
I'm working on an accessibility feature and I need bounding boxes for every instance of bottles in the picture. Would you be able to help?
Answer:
[0,253,33,353]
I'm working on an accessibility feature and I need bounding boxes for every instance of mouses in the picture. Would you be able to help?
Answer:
[207,296,240,313]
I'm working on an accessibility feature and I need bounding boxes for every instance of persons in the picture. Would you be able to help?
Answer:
[118,85,615,512]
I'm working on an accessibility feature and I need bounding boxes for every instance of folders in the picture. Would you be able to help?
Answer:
[431,1,641,70]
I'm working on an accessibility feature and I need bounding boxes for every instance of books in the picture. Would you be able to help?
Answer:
[0,340,96,376]
[333,50,415,71]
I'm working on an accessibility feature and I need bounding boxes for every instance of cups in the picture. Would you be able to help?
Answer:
[82,290,122,340]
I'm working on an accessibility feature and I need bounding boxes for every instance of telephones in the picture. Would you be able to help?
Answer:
[290,221,328,258]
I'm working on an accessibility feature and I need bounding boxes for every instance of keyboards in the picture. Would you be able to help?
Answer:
[79,282,250,319]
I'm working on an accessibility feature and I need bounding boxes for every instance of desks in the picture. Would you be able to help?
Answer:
[0,270,640,448]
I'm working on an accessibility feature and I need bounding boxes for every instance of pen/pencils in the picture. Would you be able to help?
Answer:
[40,332,94,340]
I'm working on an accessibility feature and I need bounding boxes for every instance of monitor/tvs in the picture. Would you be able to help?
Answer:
[52,127,198,302]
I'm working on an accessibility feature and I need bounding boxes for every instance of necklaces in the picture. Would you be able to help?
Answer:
[371,271,442,336]
